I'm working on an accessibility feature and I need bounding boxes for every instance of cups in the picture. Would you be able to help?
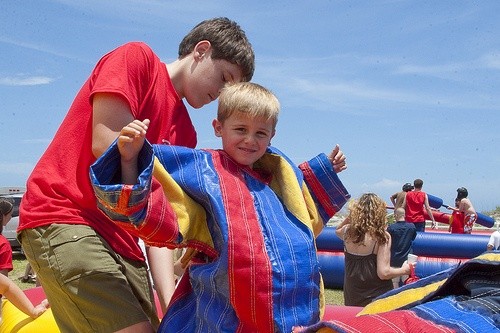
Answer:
[408,254,418,265]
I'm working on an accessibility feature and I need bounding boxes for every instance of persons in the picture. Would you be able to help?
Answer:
[0,272,49,319]
[21,264,36,282]
[402,179,436,232]
[17,17,258,332]
[335,193,417,308]
[448,187,478,234]
[386,208,417,289]
[448,197,466,234]
[89,82,351,333]
[390,183,415,215]
[0,201,15,298]
[487,224,500,251]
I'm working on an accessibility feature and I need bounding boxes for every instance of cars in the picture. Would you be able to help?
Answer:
[0,194,24,251]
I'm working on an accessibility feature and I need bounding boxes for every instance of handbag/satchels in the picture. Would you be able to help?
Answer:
[404,263,420,285]
